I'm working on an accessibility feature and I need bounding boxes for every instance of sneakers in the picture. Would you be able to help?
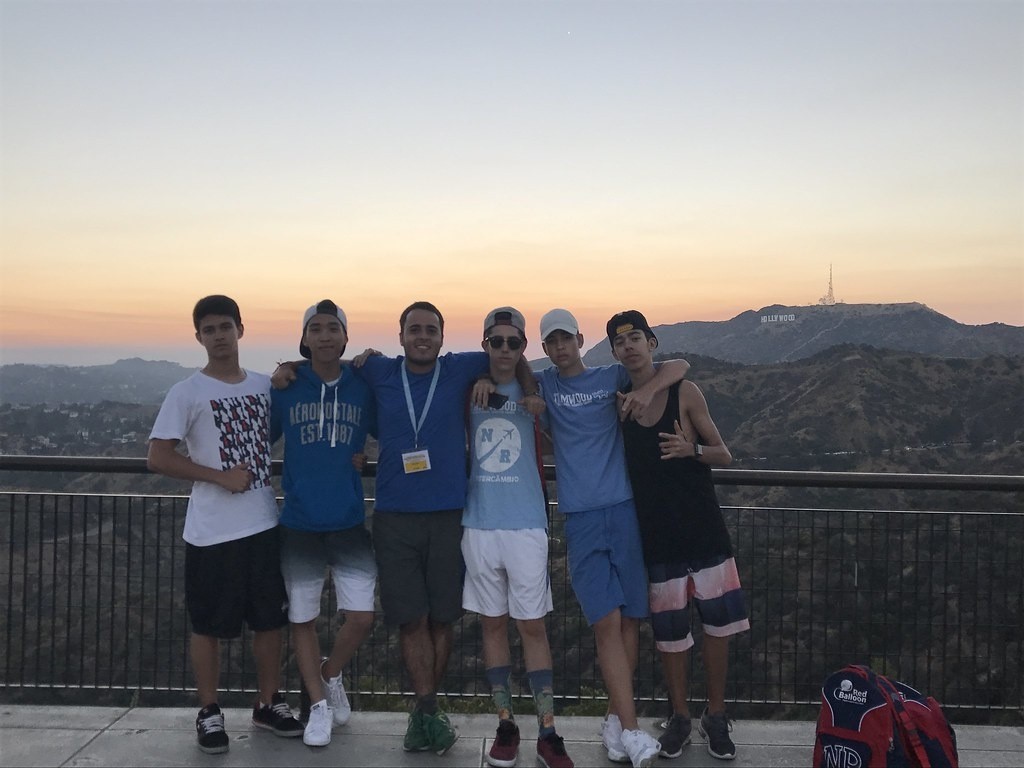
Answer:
[422,708,459,756]
[403,710,431,752]
[697,707,737,759]
[252,693,305,736]
[196,703,230,753]
[602,714,630,762]
[303,699,333,746]
[487,721,520,768]
[536,733,574,768]
[657,714,692,758]
[319,660,351,726]
[619,729,661,768]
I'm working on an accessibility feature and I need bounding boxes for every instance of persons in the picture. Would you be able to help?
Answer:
[271,301,547,755]
[146,295,367,754]
[269,295,376,747]
[350,307,750,768]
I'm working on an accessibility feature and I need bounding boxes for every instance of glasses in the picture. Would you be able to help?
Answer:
[485,335,521,350]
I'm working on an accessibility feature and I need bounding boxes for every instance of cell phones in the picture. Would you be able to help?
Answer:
[475,391,509,410]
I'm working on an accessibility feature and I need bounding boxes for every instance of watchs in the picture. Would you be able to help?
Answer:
[525,391,540,397]
[691,441,703,458]
[477,373,491,380]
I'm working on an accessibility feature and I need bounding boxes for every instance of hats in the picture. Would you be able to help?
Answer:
[540,309,579,340]
[606,310,659,349]
[482,307,525,332]
[299,300,348,359]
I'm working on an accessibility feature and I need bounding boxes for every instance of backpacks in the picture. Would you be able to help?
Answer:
[811,664,958,768]
